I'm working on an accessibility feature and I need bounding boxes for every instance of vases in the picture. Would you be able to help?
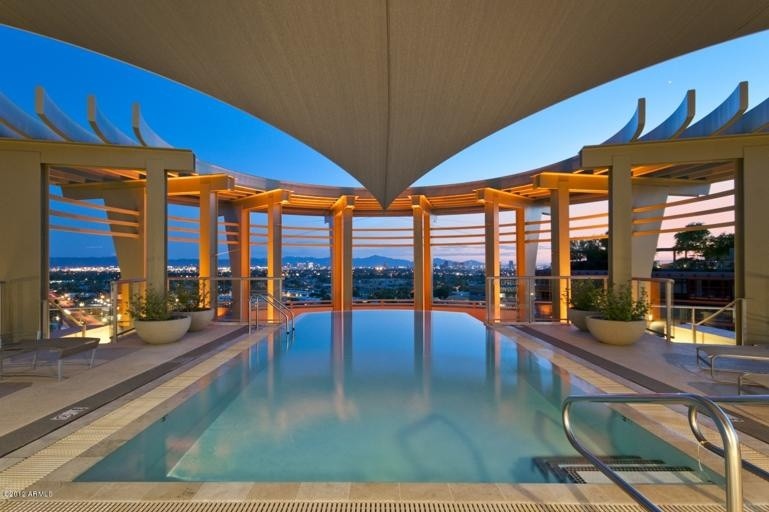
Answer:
[535,300,553,316]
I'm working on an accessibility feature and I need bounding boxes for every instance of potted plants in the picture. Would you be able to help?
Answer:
[127,277,215,345]
[560,279,656,344]
